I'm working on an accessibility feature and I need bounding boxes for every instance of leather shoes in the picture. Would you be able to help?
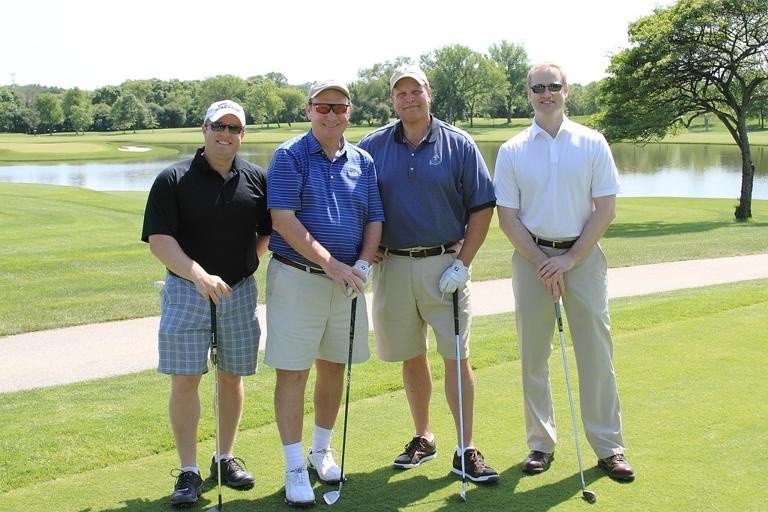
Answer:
[597,452,636,482]
[522,450,555,473]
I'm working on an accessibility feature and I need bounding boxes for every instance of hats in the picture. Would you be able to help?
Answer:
[309,78,350,101]
[204,98,247,127]
[389,63,430,92]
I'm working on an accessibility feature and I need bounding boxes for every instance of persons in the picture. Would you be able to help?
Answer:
[141,99,274,506]
[355,62,500,485]
[268,80,386,504]
[492,64,634,481]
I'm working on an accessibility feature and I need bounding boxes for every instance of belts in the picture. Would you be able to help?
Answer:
[378,243,463,259]
[530,236,581,250]
[271,250,325,275]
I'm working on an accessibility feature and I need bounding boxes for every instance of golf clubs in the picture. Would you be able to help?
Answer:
[206,296,222,512]
[322,297,357,505]
[552,283,596,504]
[451,288,467,500]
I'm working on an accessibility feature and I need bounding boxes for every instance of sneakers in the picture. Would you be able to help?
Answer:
[168,466,204,506]
[393,432,437,471]
[210,456,255,488]
[451,444,500,483]
[283,466,316,506]
[307,449,345,482]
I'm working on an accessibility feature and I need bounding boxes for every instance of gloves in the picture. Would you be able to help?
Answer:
[438,259,470,294]
[346,260,371,300]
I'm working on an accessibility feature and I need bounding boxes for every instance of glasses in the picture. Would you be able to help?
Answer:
[309,103,350,114]
[529,83,563,94]
[205,121,244,134]
